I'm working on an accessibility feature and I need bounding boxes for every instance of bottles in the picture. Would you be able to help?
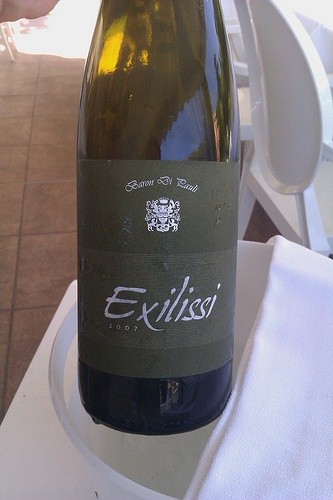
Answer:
[73,0,242,437]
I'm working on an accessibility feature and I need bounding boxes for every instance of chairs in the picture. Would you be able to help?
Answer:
[222,1,333,257]
